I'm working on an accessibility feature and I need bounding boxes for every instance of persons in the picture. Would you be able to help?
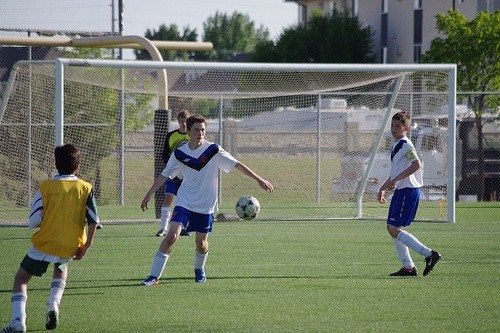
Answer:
[377,112,442,278]
[141,113,274,287]
[0,142,103,333]
[155,110,193,237]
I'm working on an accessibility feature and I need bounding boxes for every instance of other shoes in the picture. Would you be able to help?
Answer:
[180,229,190,236]
[156,228,167,236]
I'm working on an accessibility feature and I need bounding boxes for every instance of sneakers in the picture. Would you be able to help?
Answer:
[194,267,207,284]
[423,249,442,277]
[0,321,26,333]
[141,275,159,286]
[389,266,418,276]
[44,308,58,330]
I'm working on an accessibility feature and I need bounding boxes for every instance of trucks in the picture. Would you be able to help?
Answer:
[333,112,500,203]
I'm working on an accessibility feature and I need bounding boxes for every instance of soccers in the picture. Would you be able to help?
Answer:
[235,195,261,221]
